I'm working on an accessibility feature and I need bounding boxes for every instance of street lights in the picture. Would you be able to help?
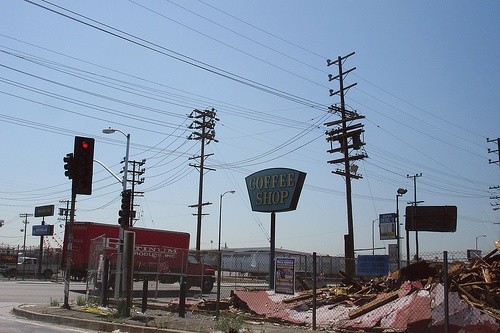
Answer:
[102,127,131,191]
[218,190,236,271]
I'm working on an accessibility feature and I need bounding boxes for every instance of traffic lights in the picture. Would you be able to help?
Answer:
[117,189,131,226]
[63,152,74,179]
[72,135,95,196]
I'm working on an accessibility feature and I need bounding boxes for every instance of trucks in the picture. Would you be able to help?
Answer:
[61,222,216,295]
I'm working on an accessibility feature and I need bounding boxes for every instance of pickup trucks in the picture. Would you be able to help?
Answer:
[0,257,60,280]
